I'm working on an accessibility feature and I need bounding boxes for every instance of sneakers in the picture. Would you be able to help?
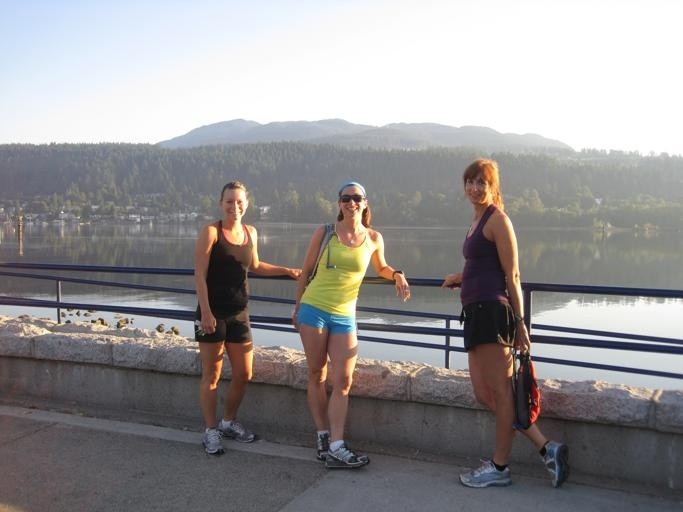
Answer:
[316,431,330,461]
[325,443,369,469]
[202,426,226,455]
[459,458,513,489]
[539,440,570,488]
[218,421,256,443]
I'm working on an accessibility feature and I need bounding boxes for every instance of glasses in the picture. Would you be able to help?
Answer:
[340,195,366,203]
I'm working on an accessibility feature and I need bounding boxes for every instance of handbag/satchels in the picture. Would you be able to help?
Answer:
[511,344,541,431]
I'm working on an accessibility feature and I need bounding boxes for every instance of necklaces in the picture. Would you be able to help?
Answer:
[340,229,365,244]
[467,224,475,232]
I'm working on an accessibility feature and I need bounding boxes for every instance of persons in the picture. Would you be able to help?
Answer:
[441,160,569,488]
[292,181,411,469]
[194,180,302,456]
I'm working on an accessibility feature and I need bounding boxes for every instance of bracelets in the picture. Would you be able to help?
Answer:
[393,270,404,280]
